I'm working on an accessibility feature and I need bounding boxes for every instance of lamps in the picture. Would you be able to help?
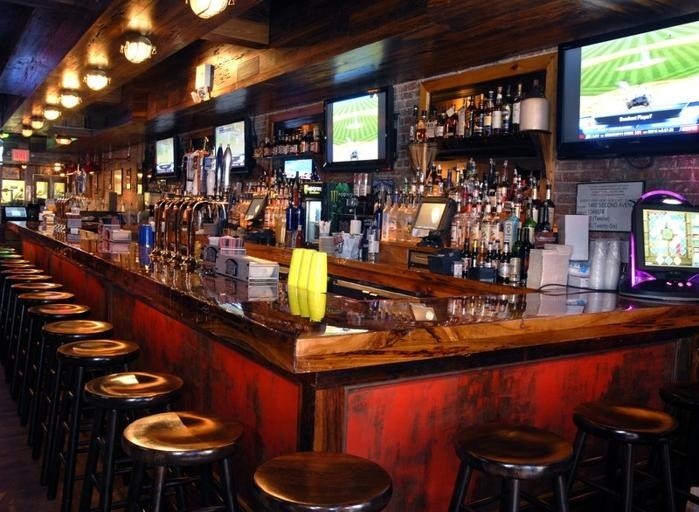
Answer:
[119,34,158,64]
[286,247,328,324]
[82,68,112,91]
[184,0,234,21]
[43,107,62,121]
[58,90,84,109]
[30,115,44,130]
[20,125,33,138]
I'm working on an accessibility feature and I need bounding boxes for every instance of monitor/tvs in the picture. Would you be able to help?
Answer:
[411,197,457,248]
[213,116,252,174]
[323,85,395,173]
[634,204,699,293]
[3,206,29,221]
[155,135,180,179]
[243,195,268,228]
[555,11,699,161]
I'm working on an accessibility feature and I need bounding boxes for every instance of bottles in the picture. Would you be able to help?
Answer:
[229,123,382,264]
[137,223,154,247]
[383,79,557,288]
[308,251,328,293]
[138,248,156,273]
[342,293,529,327]
[52,196,70,244]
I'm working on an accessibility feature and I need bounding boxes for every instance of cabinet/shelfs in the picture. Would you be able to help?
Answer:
[379,52,559,274]
[226,102,326,245]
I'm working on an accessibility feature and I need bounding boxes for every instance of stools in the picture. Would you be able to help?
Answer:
[19,303,90,444]
[11,290,75,413]
[123,411,242,511]
[78,370,184,511]
[47,340,141,511]
[1,246,63,379]
[448,427,575,512]
[566,402,680,512]
[30,319,114,485]
[251,452,393,512]
[654,385,699,484]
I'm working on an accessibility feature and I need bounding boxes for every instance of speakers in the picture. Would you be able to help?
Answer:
[84,103,110,130]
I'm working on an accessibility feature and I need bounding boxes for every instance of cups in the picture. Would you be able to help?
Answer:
[583,292,616,315]
[286,248,305,287]
[287,288,302,316]
[589,239,621,291]
[297,250,318,289]
[296,288,311,317]
[307,293,328,323]
